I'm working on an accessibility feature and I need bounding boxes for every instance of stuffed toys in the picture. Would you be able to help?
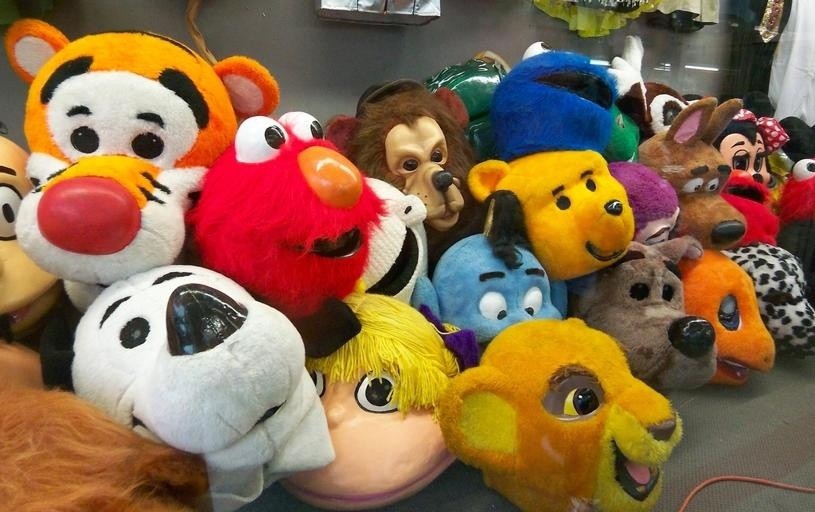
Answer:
[0,16,814,511]
